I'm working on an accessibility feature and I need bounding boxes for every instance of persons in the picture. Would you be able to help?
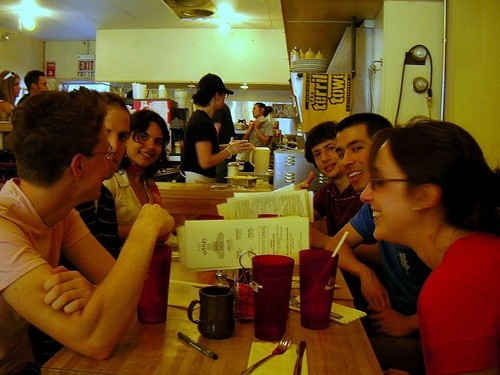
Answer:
[360,116,500,375]
[176,73,254,185]
[0,70,175,375]
[295,113,432,375]
[242,102,274,172]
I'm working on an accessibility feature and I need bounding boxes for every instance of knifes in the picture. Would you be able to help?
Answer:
[293,340,307,375]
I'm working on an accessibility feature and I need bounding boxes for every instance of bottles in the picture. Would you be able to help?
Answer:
[291,47,322,61]
[214,270,229,286]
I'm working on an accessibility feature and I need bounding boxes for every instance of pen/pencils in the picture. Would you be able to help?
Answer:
[177,332,219,360]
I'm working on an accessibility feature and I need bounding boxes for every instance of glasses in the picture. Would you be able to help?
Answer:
[85,145,117,160]
[219,93,229,98]
[368,176,410,188]
[3,71,16,80]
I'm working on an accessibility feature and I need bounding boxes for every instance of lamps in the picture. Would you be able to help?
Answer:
[188,82,196,90]
[240,82,249,90]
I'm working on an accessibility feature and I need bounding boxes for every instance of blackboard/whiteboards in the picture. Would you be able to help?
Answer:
[94,28,293,85]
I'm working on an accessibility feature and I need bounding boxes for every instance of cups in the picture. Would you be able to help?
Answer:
[174,141,181,154]
[251,254,295,341]
[187,285,236,340]
[298,249,339,330]
[228,162,239,177]
[136,245,172,323]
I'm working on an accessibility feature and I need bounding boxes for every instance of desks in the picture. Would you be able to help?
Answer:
[41,259,383,375]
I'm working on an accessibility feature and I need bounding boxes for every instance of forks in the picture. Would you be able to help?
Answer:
[240,333,294,375]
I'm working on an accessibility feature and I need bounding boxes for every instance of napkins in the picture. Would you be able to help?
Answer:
[169,279,213,309]
[289,294,368,325]
[247,342,309,375]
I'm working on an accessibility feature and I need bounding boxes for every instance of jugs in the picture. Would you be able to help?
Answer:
[249,147,270,175]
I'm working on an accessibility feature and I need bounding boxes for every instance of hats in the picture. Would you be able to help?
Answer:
[197,73,234,95]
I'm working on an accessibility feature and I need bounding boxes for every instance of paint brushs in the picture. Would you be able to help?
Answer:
[293,340,307,375]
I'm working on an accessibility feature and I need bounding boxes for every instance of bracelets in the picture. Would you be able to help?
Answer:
[224,148,232,159]
